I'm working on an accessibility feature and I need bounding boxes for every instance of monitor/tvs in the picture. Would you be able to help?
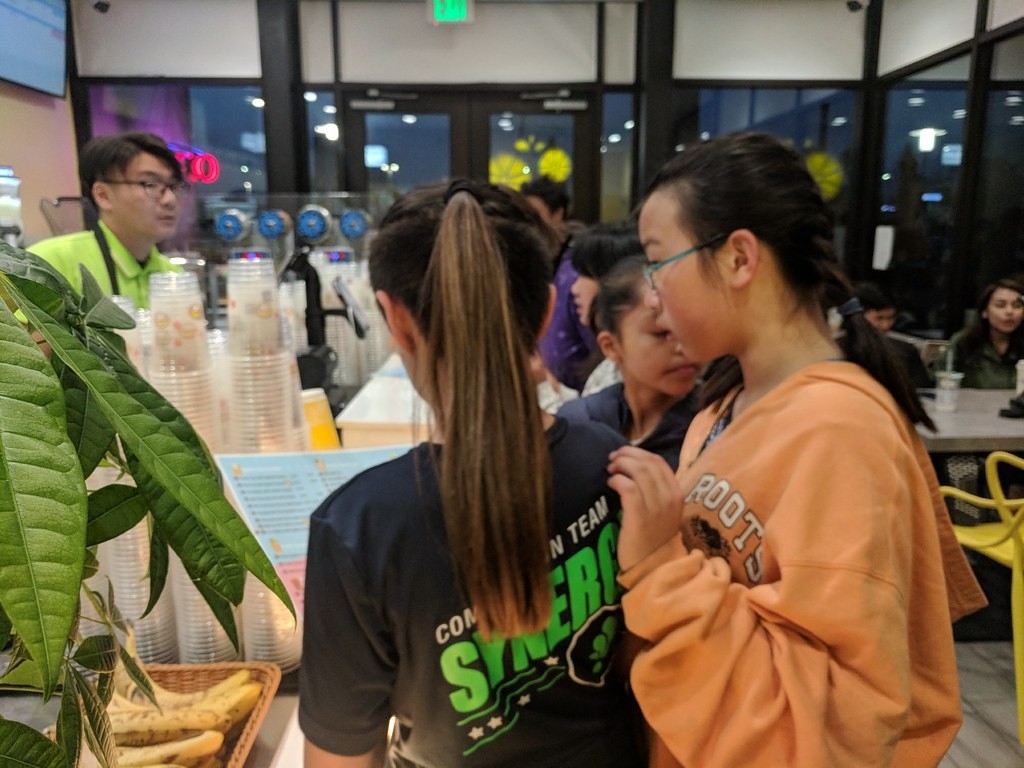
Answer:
[0,0,70,99]
[333,277,369,340]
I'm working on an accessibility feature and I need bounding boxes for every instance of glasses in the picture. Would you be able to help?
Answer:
[642,232,726,289]
[103,179,192,200]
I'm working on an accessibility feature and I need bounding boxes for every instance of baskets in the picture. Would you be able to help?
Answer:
[40,660,281,768]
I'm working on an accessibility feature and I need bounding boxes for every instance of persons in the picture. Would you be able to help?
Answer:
[13,132,192,363]
[298,131,1024,768]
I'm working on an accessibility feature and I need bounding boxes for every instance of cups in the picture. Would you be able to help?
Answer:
[1016,359,1024,398]
[935,373,964,414]
[70,258,391,680]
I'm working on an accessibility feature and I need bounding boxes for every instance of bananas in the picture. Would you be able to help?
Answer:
[43,622,264,768]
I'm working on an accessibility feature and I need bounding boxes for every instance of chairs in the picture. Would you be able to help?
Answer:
[937,452,1023,744]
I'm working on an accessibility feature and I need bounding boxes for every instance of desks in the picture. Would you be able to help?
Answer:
[909,365,1023,539]
[337,298,585,450]
